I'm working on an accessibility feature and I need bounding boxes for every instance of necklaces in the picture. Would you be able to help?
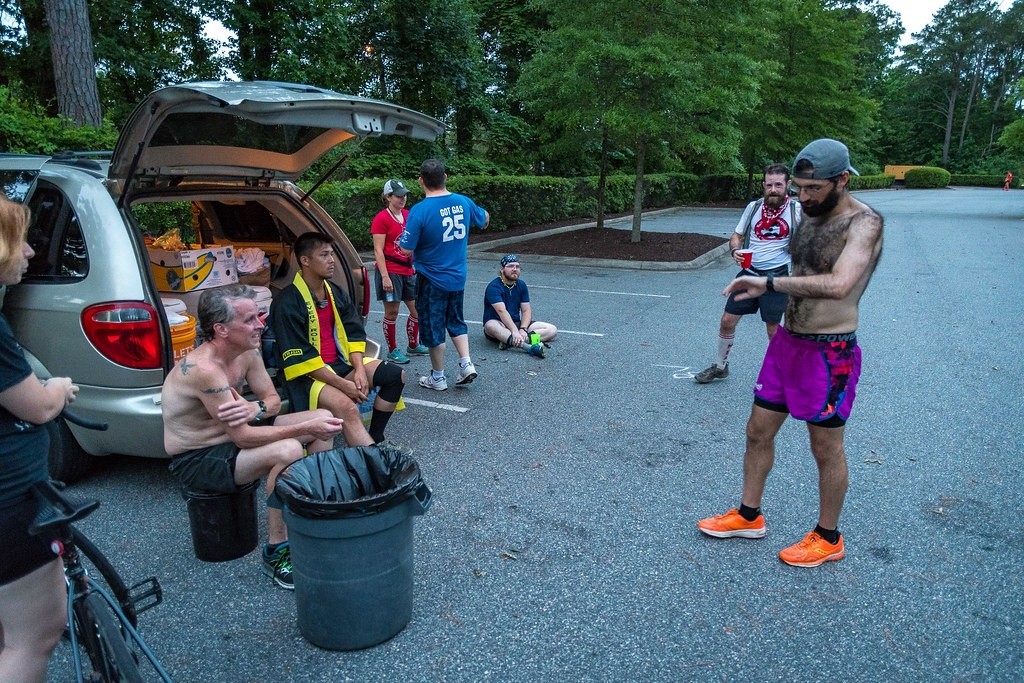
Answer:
[500,276,516,296]
[388,207,404,231]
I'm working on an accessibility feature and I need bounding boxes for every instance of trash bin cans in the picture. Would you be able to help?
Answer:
[275,444,433,652]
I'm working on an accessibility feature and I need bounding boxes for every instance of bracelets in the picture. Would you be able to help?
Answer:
[382,273,388,277]
[766,273,776,293]
[731,249,740,257]
[251,400,267,423]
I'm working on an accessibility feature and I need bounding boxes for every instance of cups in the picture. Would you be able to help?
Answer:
[531,334,541,345]
[385,290,394,302]
[738,249,753,268]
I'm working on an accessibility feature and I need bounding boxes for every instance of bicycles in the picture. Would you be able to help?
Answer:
[26,408,175,683]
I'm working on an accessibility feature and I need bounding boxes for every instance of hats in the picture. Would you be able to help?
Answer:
[500,254,519,266]
[384,179,413,197]
[790,138,860,179]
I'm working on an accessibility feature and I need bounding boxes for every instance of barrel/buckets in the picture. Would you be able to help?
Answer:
[179,478,262,562]
[159,312,197,366]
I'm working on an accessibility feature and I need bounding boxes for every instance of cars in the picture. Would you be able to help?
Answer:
[1,77,454,487]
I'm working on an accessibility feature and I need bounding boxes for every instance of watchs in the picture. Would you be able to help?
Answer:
[520,326,527,332]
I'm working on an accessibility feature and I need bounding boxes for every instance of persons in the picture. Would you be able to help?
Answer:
[0,190,79,683]
[269,232,405,455]
[697,138,884,567]
[370,179,430,364]
[400,159,489,391]
[482,254,557,358]
[1002,170,1012,191]
[695,164,802,382]
[162,284,344,590]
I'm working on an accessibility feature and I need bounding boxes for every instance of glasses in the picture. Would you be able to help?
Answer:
[790,180,831,194]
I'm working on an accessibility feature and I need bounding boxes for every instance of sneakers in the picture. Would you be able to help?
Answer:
[261,540,297,589]
[406,344,431,356]
[694,361,730,384]
[698,508,766,538]
[498,341,508,350]
[419,370,448,391]
[529,342,545,359]
[455,362,478,385]
[386,348,410,364]
[779,530,844,568]
[377,431,413,457]
